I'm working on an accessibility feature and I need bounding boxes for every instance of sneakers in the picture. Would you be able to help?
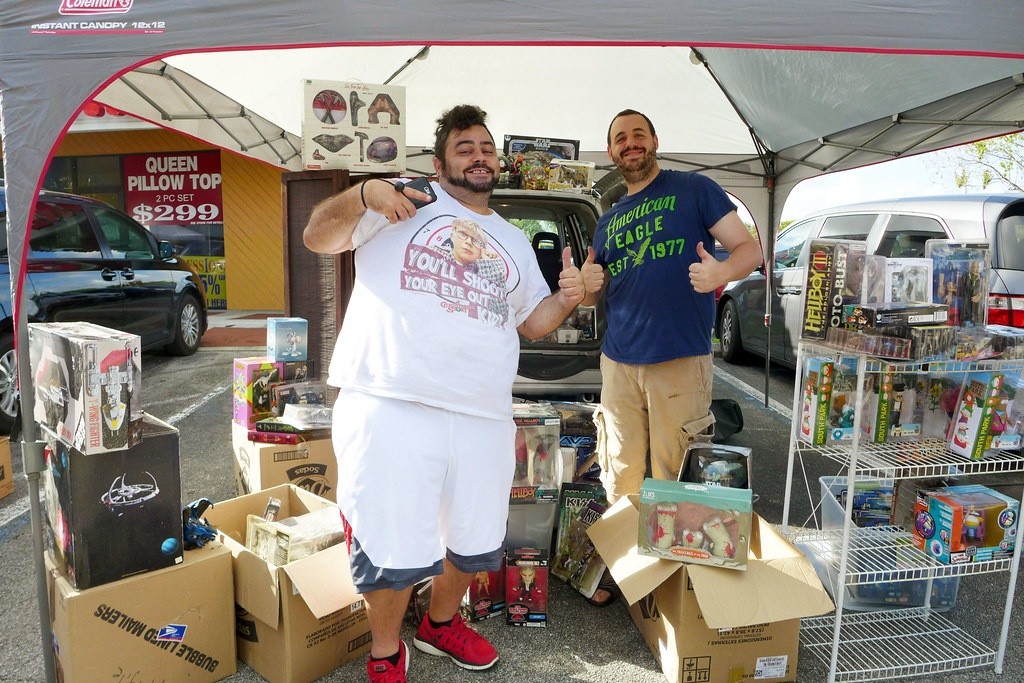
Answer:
[413,610,499,670]
[367,639,409,683]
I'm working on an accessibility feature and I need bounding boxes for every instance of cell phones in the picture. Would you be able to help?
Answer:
[384,175,438,220]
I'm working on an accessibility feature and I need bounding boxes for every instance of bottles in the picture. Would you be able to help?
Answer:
[263,497,282,522]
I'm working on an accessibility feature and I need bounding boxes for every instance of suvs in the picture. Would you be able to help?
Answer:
[0,177,210,433]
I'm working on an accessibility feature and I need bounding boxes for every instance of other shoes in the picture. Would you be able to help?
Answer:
[586,566,620,608]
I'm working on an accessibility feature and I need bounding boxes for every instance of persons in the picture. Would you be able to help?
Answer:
[831,394,854,427]
[889,384,904,428]
[427,216,497,273]
[303,104,584,683]
[580,108,762,606]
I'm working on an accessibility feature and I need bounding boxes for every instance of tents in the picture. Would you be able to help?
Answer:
[0,0,1024,683]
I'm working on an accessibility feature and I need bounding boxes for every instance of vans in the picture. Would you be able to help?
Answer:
[712,194,1024,366]
[492,189,606,404]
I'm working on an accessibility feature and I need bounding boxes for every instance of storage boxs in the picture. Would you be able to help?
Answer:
[798,239,1024,460]
[498,134,595,195]
[301,77,406,172]
[0,434,16,501]
[555,307,596,344]
[817,476,962,612]
[912,484,1020,564]
[25,316,835,683]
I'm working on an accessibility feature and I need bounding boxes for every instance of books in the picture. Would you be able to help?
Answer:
[248,417,329,444]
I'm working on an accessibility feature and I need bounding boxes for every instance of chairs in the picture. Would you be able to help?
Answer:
[532,232,563,293]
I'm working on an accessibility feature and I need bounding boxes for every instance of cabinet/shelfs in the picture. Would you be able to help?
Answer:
[780,341,1023,683]
